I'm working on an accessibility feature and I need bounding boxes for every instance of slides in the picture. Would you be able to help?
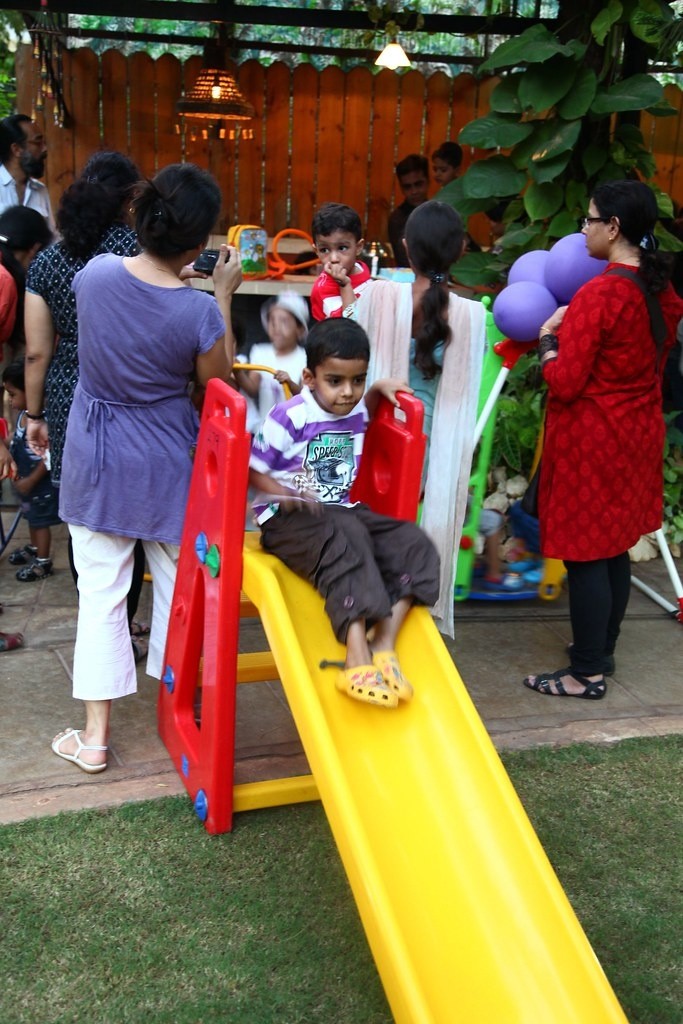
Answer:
[230,531,627,1023]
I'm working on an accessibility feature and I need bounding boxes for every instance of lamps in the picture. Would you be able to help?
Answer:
[174,20,256,140]
[375,37,412,70]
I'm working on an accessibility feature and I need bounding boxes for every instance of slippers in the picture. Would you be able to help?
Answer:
[373,650,413,699]
[335,664,399,711]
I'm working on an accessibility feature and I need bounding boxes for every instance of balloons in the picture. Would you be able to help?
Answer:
[493,233,609,342]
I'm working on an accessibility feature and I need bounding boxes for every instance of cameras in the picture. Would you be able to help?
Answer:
[193,249,230,275]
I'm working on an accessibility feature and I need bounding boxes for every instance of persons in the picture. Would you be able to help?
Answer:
[232,196,526,709]
[0,113,245,774]
[521,178,683,700]
[387,140,483,283]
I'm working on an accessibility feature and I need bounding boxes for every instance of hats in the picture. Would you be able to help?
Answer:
[484,194,528,224]
[261,290,310,346]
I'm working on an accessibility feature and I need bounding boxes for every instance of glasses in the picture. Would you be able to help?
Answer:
[21,136,47,148]
[580,216,612,232]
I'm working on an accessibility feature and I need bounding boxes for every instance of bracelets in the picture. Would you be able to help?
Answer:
[539,326,553,334]
[24,411,47,419]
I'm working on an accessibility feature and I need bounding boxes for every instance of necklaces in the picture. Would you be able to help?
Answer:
[135,255,176,277]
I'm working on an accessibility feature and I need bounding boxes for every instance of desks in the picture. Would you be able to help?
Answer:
[190,235,318,295]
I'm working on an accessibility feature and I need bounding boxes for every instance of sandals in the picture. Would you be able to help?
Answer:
[16,557,53,581]
[567,642,616,676]
[51,728,107,773]
[523,664,608,700]
[8,544,37,565]
[130,635,148,663]
[131,619,151,635]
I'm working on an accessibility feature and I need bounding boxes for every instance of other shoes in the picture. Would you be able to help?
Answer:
[0,632,23,651]
[481,570,526,591]
